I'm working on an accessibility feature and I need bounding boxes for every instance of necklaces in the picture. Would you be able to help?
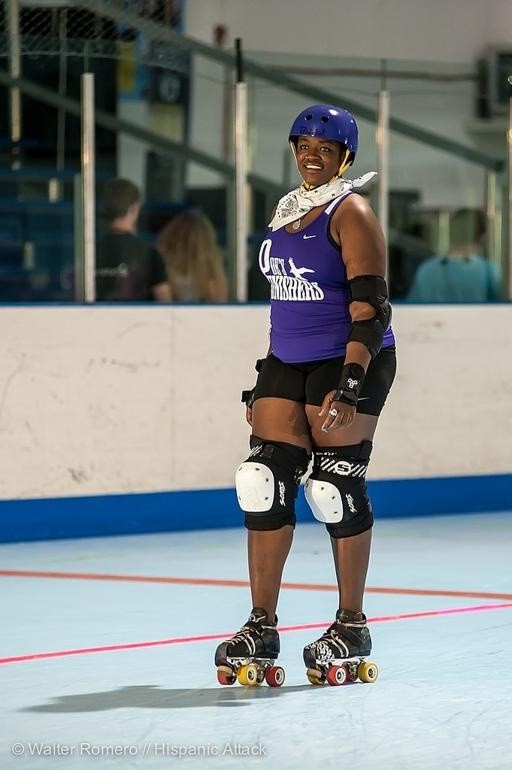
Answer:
[290,218,305,231]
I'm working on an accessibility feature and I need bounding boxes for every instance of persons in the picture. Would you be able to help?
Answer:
[87,174,168,307]
[209,102,398,664]
[404,203,509,307]
[152,202,226,302]
[384,213,441,309]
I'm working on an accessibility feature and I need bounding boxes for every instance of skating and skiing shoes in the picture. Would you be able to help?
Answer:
[304,609,379,685]
[215,607,285,686]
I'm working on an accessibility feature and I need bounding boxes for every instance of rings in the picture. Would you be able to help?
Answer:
[327,406,340,419]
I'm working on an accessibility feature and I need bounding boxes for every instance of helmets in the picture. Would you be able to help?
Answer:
[288,105,358,166]
